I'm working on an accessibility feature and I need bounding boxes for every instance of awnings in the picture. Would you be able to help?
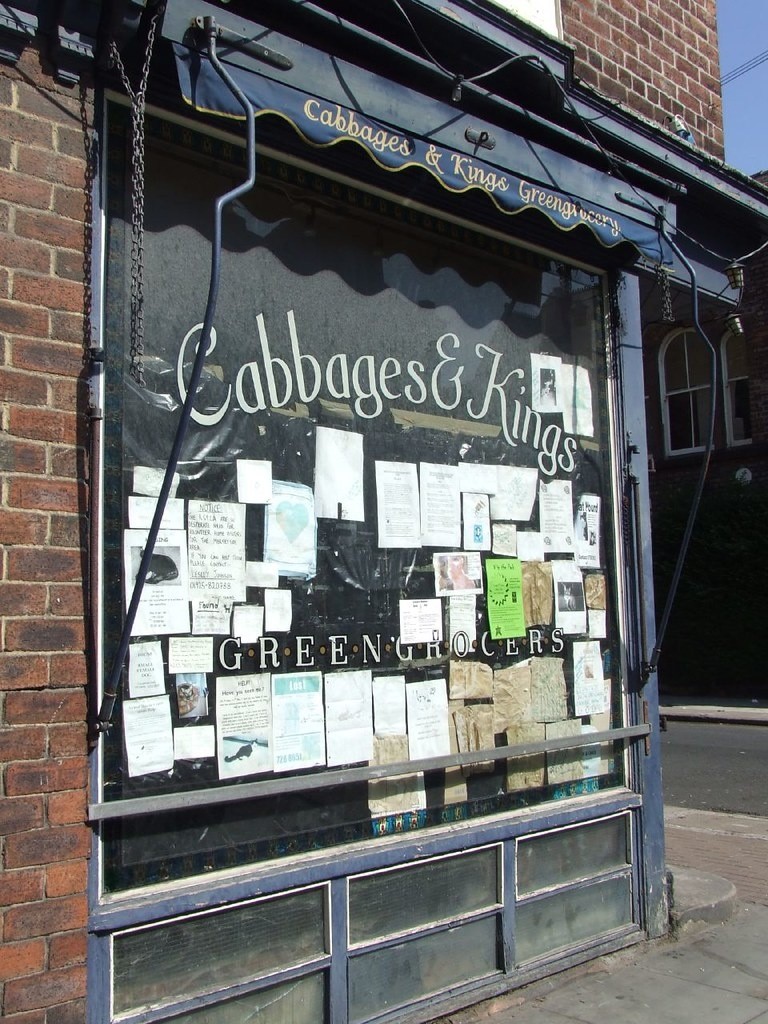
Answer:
[174,43,675,268]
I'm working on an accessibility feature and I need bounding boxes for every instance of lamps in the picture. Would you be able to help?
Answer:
[723,256,745,288]
[723,311,745,337]
[660,114,690,141]
